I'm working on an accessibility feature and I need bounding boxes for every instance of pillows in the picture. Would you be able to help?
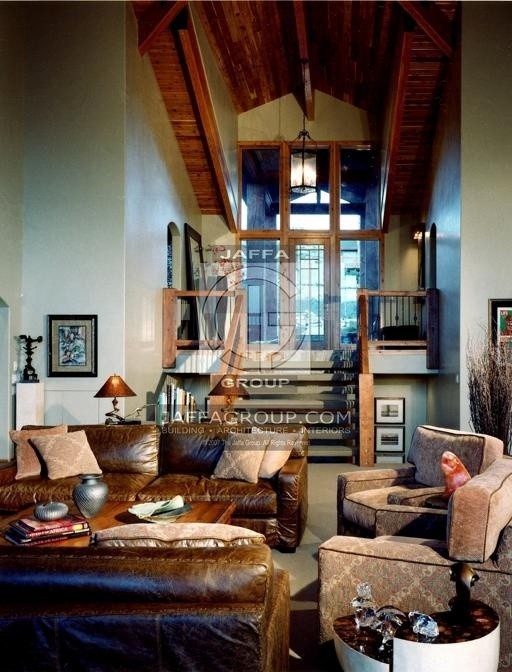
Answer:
[89,523,267,547]
[29,430,103,480]
[250,426,298,480]
[5,424,68,480]
[211,432,270,484]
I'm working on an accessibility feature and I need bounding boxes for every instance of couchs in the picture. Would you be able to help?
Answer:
[0,547,290,672]
[318,455,512,672]
[337,426,504,538]
[0,423,309,553]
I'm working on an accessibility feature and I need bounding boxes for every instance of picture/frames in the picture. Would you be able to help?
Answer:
[374,397,405,424]
[374,454,405,464]
[46,315,98,377]
[183,222,207,340]
[374,426,405,452]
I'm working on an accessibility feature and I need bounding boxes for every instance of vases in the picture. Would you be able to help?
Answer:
[73,474,108,519]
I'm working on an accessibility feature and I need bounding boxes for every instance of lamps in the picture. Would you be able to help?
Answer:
[488,298,512,366]
[291,113,318,194]
[208,375,249,423]
[94,373,137,425]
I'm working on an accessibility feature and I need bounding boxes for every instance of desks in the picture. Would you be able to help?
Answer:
[331,595,500,672]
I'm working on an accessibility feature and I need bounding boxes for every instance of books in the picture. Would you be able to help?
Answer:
[3,513,92,546]
[158,383,198,424]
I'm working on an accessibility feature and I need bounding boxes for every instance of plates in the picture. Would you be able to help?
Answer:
[128,499,192,523]
